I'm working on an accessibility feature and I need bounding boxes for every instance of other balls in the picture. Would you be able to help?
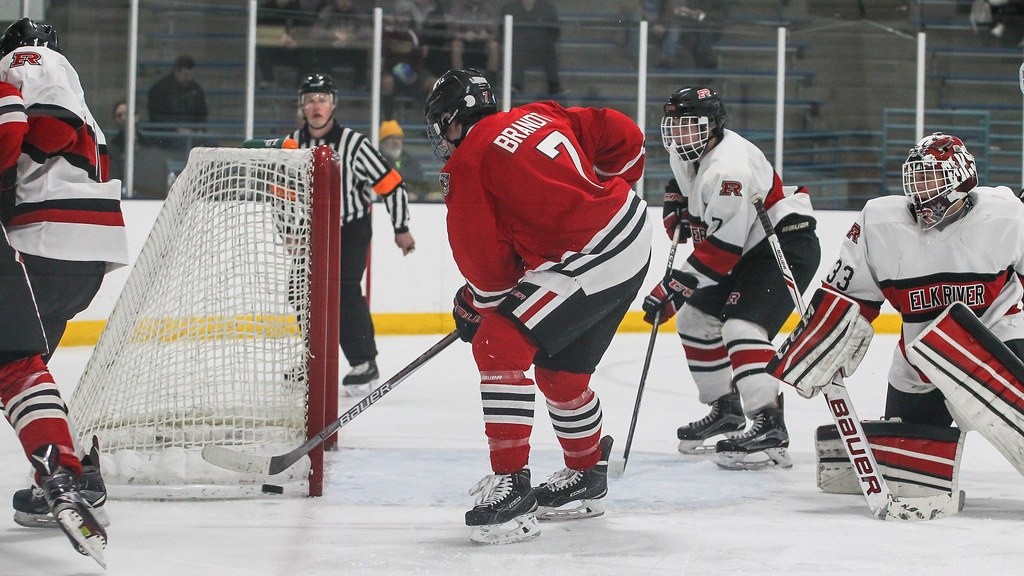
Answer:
[262,484,284,494]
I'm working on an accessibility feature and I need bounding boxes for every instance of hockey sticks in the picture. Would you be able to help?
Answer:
[750,196,966,520]
[202,327,462,476]
[606,220,682,480]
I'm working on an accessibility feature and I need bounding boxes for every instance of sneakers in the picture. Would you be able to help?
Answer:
[464,468,542,545]
[342,357,381,397]
[12,434,111,528]
[715,392,793,471]
[677,381,748,455]
[531,435,614,523]
[31,443,109,571]
[282,360,309,390]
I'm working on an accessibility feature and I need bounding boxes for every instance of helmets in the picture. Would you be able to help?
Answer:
[423,68,498,137]
[297,72,338,96]
[904,131,979,222]
[0,17,64,61]
[663,86,726,144]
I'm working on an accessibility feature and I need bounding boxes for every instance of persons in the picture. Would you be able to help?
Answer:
[271,71,417,400]
[376,116,426,203]
[795,132,1024,500]
[643,84,821,471]
[149,55,220,150]
[0,18,130,571]
[629,0,729,71]
[426,66,654,546]
[992,1,1024,48]
[258,0,573,113]
[106,100,157,183]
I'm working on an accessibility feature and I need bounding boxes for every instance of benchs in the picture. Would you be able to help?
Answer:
[45,8,865,212]
[915,8,1023,190]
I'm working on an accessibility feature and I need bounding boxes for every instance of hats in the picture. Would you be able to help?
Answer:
[379,119,404,141]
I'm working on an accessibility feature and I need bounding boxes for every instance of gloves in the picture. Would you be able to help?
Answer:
[663,179,692,244]
[642,269,699,326]
[453,283,481,344]
